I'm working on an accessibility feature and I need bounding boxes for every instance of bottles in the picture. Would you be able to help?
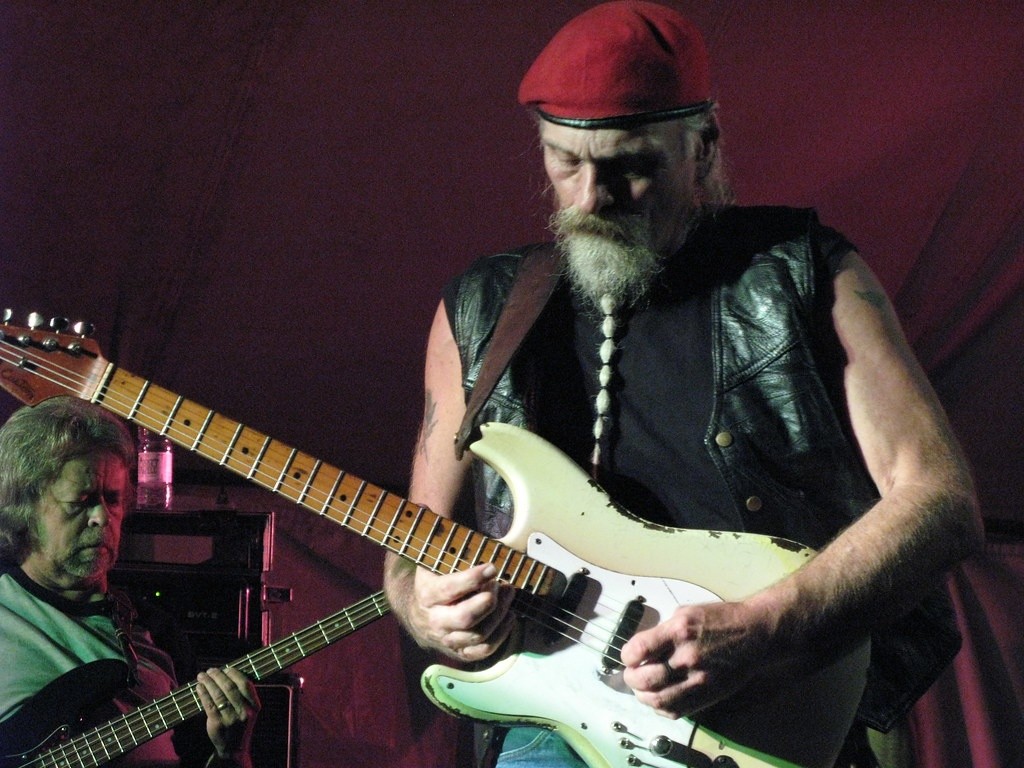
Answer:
[136,426,173,509]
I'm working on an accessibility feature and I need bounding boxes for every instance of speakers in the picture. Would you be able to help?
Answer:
[170,671,302,768]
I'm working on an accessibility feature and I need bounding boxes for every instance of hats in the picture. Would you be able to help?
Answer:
[518,0,713,130]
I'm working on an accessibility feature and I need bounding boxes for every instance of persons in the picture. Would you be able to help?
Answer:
[0,397,261,768]
[384,0,987,768]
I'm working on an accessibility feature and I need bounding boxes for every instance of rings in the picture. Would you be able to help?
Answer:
[663,659,674,676]
[217,701,232,710]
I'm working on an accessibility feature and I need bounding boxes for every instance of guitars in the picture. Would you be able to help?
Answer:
[0,307,873,767]
[2,583,391,766]
